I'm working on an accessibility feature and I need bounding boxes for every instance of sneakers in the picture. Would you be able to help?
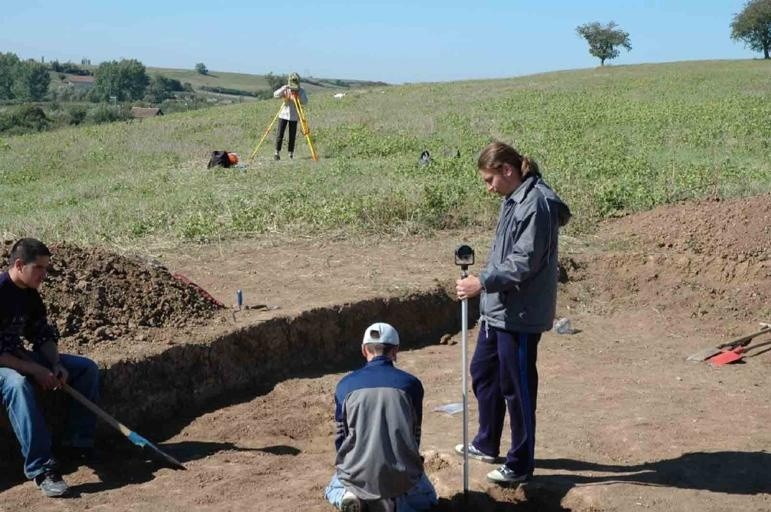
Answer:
[287,153,293,160]
[33,470,70,498]
[486,463,533,487]
[274,152,281,160]
[340,491,395,511]
[454,442,500,463]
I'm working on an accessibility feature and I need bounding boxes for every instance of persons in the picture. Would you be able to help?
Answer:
[456,141,571,485]
[325,323,439,512]
[273,72,308,160]
[0,238,106,496]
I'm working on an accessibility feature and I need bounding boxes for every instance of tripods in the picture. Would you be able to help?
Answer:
[250,93,318,162]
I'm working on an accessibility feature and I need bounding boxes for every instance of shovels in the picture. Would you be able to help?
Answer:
[686,323,771,362]
[232,290,243,323]
[705,339,771,365]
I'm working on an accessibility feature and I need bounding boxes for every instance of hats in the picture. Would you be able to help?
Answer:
[363,322,401,347]
